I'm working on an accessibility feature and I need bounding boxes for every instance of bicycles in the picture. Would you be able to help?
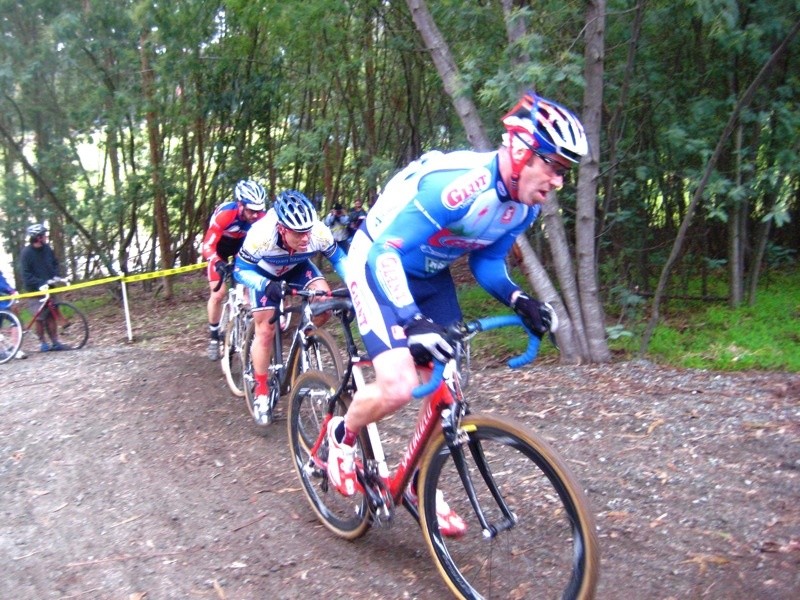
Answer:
[212,262,258,398]
[286,296,600,599]
[0,278,89,365]
[242,286,351,418]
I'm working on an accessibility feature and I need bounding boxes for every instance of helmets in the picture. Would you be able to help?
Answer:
[26,224,47,238]
[501,91,588,163]
[273,191,317,233]
[235,180,266,210]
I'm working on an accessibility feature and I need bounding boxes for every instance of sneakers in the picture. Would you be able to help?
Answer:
[326,415,359,497]
[40,344,49,352]
[206,337,220,362]
[253,382,273,427]
[14,350,28,360]
[406,469,469,539]
[50,342,70,350]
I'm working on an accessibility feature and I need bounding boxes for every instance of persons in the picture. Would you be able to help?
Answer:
[0,270,27,360]
[326,95,588,540]
[325,198,367,256]
[19,224,71,351]
[233,190,348,426]
[203,182,271,361]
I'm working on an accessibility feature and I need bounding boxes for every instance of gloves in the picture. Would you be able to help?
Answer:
[397,313,455,367]
[512,292,558,337]
[214,259,229,276]
[264,280,291,302]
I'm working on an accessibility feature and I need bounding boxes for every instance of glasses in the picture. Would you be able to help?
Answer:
[518,131,572,177]
[245,208,264,215]
